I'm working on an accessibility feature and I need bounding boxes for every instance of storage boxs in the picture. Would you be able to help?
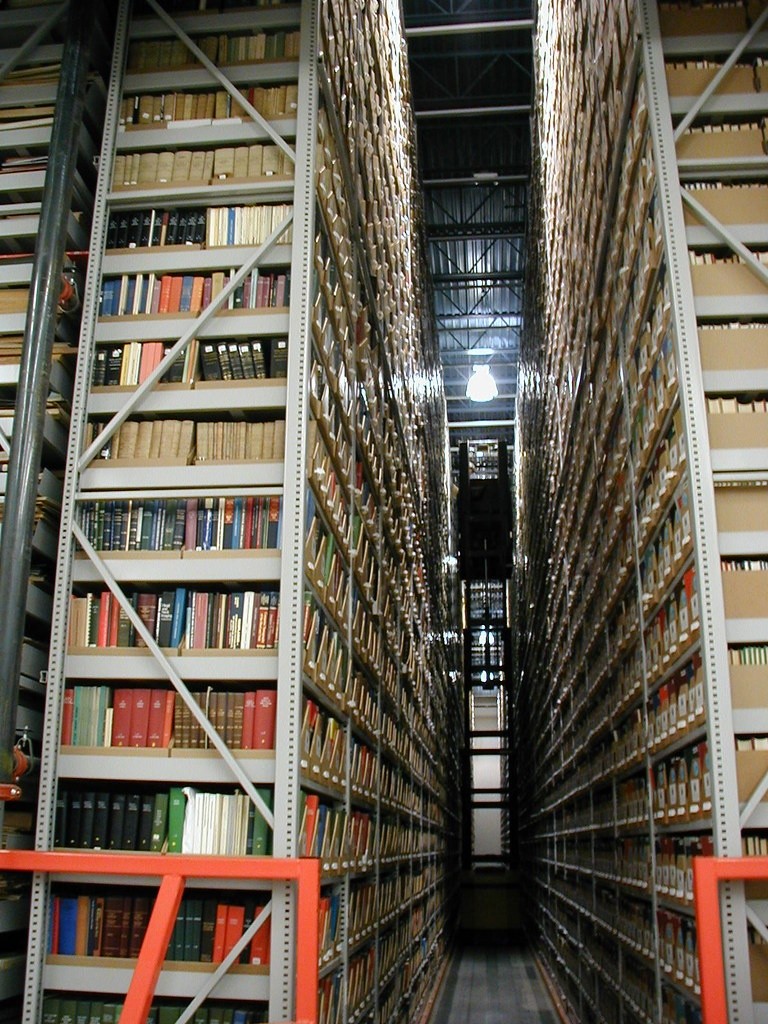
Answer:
[540,6,767,1023]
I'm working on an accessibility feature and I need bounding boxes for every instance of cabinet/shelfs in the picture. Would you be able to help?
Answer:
[504,0,768,1024]
[0,0,475,1024]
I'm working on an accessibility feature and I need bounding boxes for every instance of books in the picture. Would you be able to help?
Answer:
[0,0,477,1024]
[500,0,767,1024]
